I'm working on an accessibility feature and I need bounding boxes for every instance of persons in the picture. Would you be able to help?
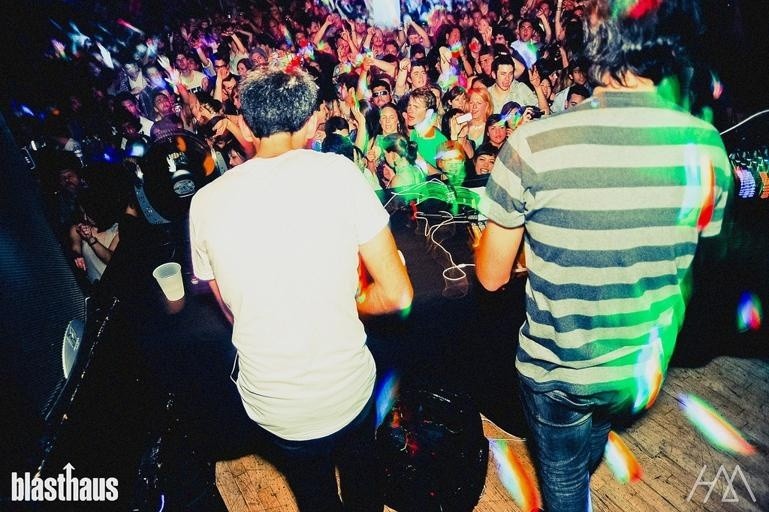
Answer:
[189,65,414,512]
[472,0,731,512]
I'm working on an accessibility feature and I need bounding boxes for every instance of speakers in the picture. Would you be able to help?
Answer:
[0,118,89,427]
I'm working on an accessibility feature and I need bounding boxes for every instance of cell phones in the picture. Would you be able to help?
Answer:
[457,113,473,123]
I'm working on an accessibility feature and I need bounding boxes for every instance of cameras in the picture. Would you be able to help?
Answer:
[525,105,544,120]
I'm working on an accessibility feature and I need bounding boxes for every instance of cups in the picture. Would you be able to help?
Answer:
[152,261,186,303]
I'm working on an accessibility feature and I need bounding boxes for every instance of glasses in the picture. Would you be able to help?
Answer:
[213,65,225,69]
[372,91,388,97]
